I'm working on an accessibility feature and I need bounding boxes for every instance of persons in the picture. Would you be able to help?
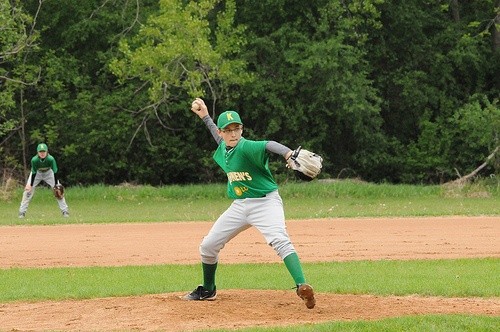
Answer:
[17,143,70,218]
[186,97,321,309]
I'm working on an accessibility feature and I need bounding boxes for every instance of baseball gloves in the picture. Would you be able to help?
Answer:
[285,147,325,182]
[54,185,64,199]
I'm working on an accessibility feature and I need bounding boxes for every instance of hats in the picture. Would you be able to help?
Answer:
[37,143,47,152]
[217,111,242,129]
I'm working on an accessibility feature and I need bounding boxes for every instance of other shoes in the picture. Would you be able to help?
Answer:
[18,212,25,218]
[63,211,69,217]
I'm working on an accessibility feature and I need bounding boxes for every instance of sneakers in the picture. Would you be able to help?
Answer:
[179,285,217,301]
[292,283,316,309]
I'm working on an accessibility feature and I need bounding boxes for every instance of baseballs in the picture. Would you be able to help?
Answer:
[192,100,201,110]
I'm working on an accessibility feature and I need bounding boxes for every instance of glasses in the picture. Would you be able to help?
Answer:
[220,125,243,135]
